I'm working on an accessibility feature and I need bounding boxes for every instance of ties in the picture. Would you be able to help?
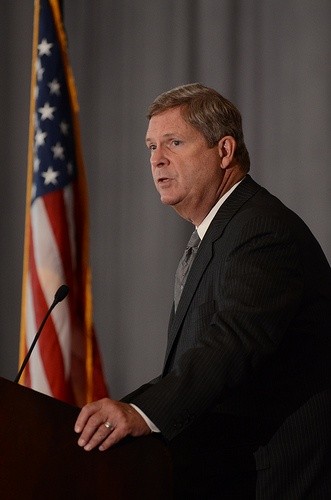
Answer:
[174,229,202,313]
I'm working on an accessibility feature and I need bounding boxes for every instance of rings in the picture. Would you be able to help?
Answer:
[103,421,115,431]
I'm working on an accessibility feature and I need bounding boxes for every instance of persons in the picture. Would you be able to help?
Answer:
[75,82,331,500]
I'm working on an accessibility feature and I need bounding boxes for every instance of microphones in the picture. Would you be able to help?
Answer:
[13,285,71,384]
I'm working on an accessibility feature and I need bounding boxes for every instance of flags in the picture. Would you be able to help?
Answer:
[19,0,111,413]
